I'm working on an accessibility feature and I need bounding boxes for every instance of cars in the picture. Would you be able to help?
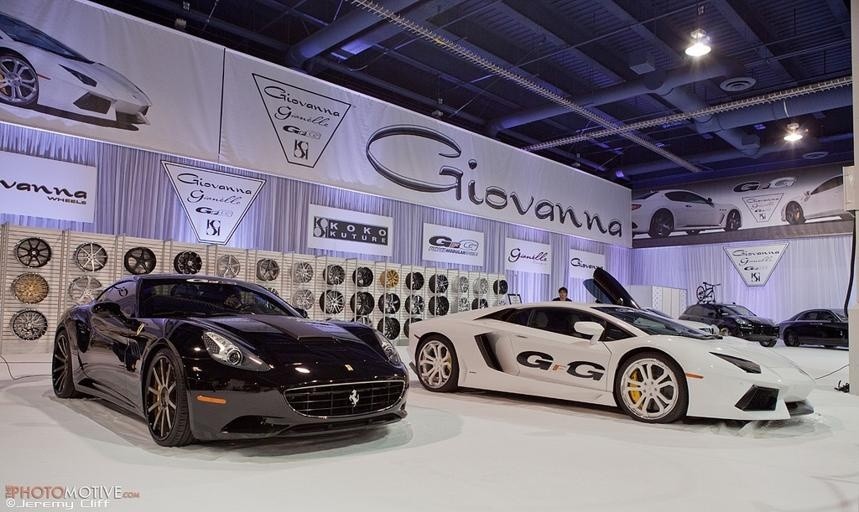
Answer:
[678,302,779,349]
[775,307,848,350]
[631,187,741,240]
[779,172,856,226]
[641,305,720,337]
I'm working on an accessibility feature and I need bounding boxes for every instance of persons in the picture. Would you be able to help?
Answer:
[552,287,572,303]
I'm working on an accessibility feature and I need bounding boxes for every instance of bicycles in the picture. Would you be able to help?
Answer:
[696,282,721,304]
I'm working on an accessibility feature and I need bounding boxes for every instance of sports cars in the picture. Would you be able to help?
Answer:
[52,270,412,450]
[408,299,815,425]
[0,11,153,130]
